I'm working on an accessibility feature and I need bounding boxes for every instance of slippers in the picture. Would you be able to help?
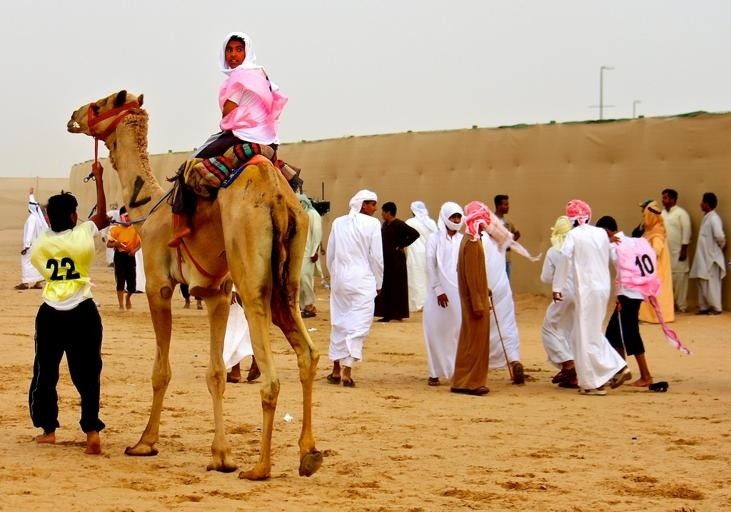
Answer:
[300,307,319,319]
[424,375,441,389]
[509,361,526,387]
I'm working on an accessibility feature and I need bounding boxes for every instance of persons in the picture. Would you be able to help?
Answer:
[595,216,653,388]
[659,190,692,312]
[14,187,49,289]
[29,162,111,454]
[294,193,323,318]
[124,248,146,293]
[552,200,631,396]
[166,32,279,248]
[494,194,520,281]
[631,199,656,237]
[540,215,580,390]
[688,193,727,315]
[424,201,464,385]
[483,211,525,384]
[105,206,142,310]
[374,202,420,323]
[637,201,675,325]
[450,201,492,395]
[404,201,439,312]
[326,190,383,387]
[180,285,203,310]
[222,284,261,383]
[103,202,120,267]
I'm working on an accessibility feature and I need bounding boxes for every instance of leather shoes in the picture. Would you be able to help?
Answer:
[326,373,341,384]
[551,367,577,384]
[343,376,356,388]
[577,388,608,396]
[246,365,261,382]
[451,384,490,396]
[226,372,238,385]
[610,369,632,390]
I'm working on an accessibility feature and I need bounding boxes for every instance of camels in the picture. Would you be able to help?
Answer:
[67,91,324,480]
